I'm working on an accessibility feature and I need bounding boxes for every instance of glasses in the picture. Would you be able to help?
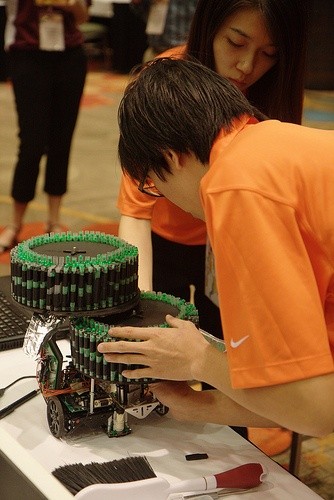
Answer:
[138,164,165,197]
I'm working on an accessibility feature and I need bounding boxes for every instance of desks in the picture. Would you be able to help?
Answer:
[0,338,327,500]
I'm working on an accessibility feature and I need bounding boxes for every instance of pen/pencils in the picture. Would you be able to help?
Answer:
[0,389,41,418]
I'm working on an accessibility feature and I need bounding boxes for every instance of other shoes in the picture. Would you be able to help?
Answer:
[46,224,67,234]
[0,223,21,248]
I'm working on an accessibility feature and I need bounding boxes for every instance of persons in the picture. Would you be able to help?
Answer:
[147,0,197,56]
[97,56,334,437]
[0,0,89,254]
[116,0,304,341]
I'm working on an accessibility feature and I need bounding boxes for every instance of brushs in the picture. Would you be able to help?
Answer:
[52,456,268,500]
